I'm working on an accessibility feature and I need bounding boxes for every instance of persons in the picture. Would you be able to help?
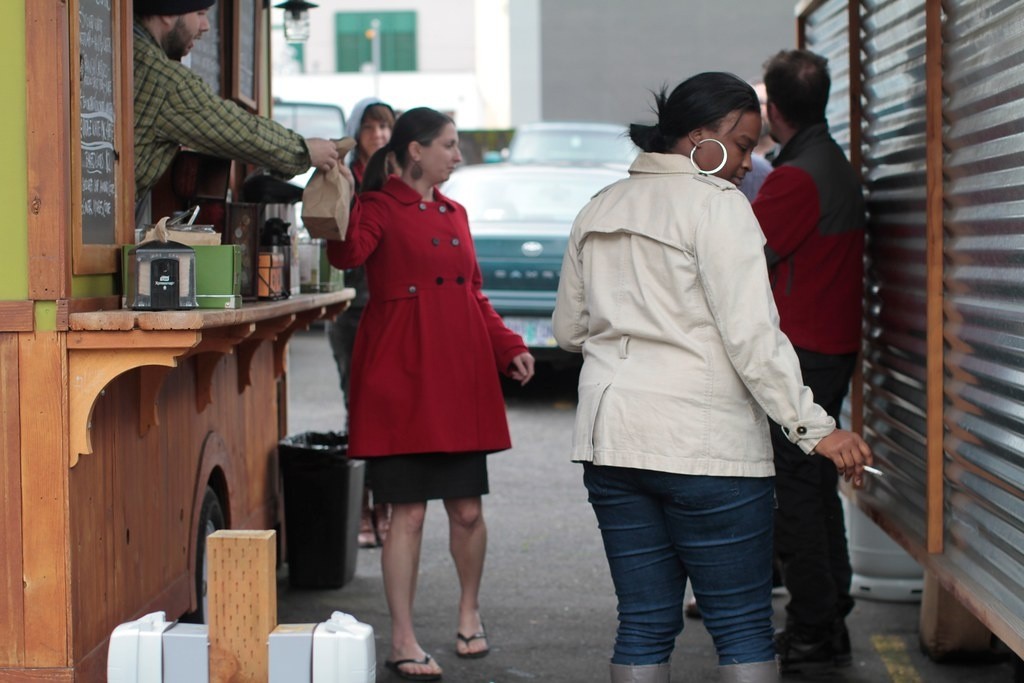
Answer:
[326,99,401,548]
[685,50,865,671]
[133,0,339,229]
[551,71,872,683]
[326,107,535,683]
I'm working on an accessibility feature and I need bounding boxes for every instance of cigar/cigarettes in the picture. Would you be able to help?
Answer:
[863,465,882,476]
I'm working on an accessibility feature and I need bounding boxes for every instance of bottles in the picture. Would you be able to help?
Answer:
[139,223,218,244]
[295,236,321,294]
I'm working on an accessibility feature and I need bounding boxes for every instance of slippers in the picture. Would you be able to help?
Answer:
[385,654,442,681]
[456,622,491,659]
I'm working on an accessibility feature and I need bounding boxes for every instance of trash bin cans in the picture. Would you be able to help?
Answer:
[278,430,364,589]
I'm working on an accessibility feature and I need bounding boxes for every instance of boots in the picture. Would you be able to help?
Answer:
[375,503,393,545]
[774,606,835,675]
[717,660,778,683]
[833,596,854,666]
[609,662,671,683]
[358,488,378,547]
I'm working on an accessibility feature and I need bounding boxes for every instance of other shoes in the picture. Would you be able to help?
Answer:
[686,597,703,619]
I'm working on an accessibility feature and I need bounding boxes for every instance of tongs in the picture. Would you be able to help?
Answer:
[165,204,200,230]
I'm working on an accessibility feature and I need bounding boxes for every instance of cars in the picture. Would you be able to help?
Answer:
[267,71,647,351]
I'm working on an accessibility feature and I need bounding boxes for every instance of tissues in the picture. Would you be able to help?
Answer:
[126,216,201,311]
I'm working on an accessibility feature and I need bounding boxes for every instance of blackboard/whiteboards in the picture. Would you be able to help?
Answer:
[68,0,125,275]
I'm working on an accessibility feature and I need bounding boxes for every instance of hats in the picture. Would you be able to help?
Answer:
[132,0,215,15]
